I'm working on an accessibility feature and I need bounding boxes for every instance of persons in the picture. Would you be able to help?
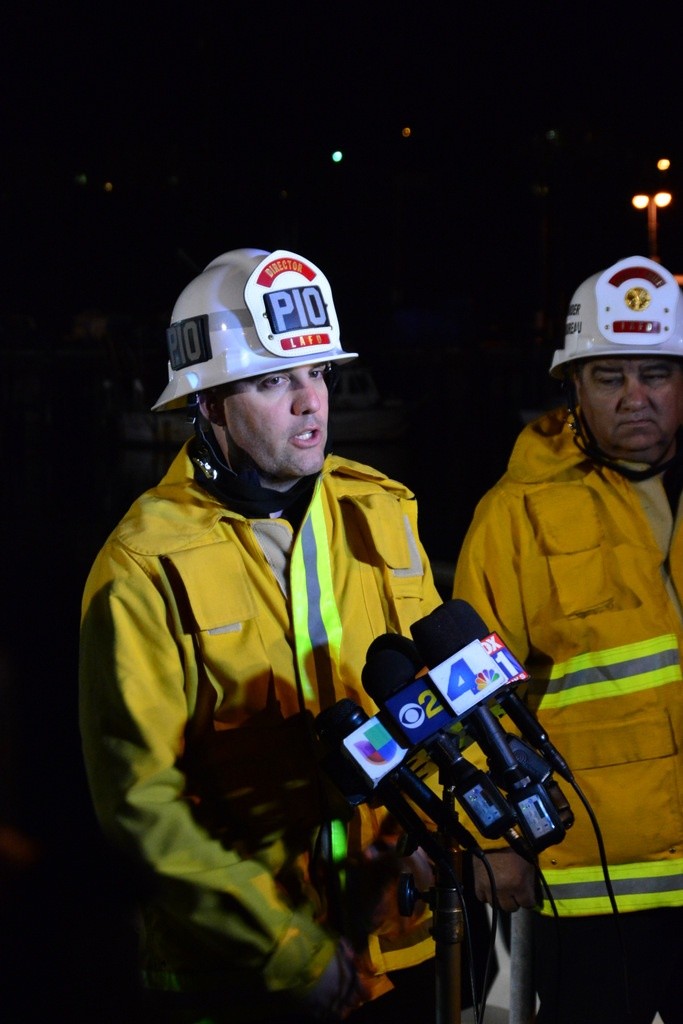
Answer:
[77,250,500,1024]
[449,257,682,1024]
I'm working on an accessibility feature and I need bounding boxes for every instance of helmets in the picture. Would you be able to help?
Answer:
[150,248,359,412]
[548,255,683,380]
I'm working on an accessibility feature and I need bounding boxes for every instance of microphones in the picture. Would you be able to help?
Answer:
[312,598,574,865]
